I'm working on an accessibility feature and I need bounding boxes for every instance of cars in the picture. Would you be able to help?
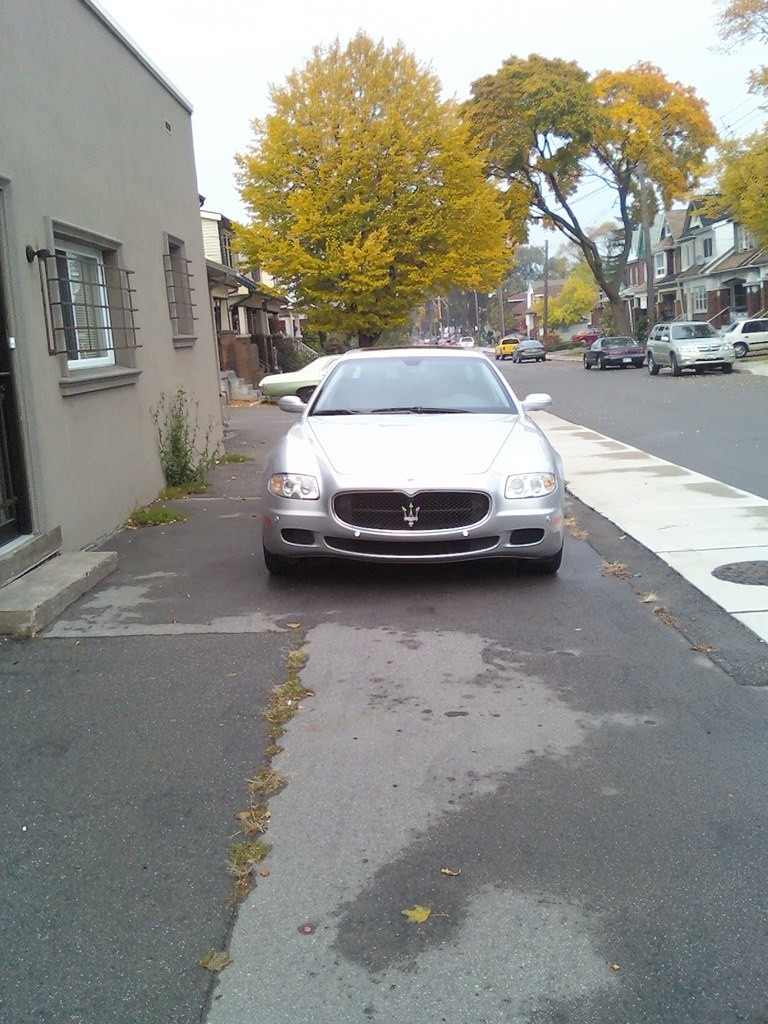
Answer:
[422,336,457,346]
[512,339,546,364]
[458,336,475,349]
[582,336,646,370]
[720,318,768,359]
[260,354,348,405]
[261,346,566,576]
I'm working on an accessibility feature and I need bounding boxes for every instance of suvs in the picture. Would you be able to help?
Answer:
[494,337,521,361]
[645,320,736,377]
